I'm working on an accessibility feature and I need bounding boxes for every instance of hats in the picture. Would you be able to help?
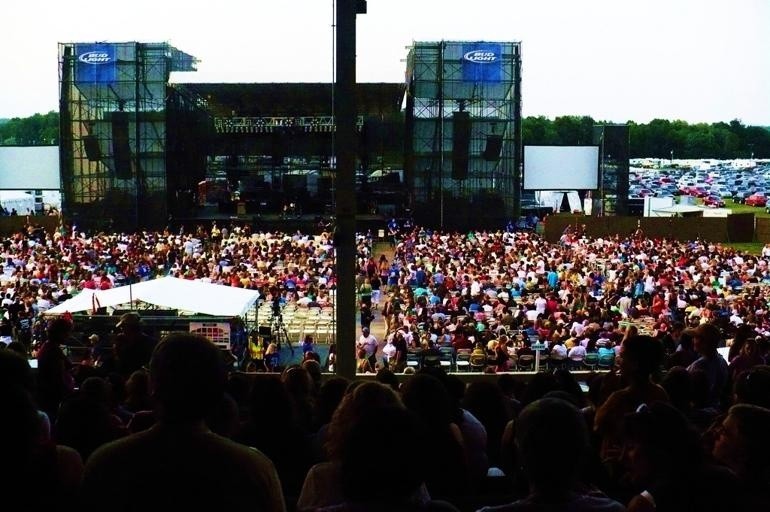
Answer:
[115,313,139,328]
[688,324,722,340]
[88,334,98,339]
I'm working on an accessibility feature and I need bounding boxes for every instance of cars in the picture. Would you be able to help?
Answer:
[598,155,770,218]
[197,154,404,202]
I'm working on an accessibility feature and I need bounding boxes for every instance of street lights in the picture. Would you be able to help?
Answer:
[747,143,755,158]
[670,148,676,160]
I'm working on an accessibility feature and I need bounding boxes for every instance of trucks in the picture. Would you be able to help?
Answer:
[0,189,35,218]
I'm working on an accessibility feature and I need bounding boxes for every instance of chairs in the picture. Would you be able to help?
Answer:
[245,302,336,345]
[390,273,768,369]
